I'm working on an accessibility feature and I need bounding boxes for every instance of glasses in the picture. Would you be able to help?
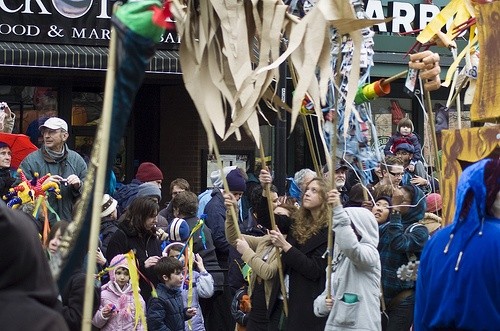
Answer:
[389,171,404,177]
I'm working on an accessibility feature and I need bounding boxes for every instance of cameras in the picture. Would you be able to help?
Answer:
[59,178,68,187]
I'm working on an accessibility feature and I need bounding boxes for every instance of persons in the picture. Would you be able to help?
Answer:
[0,101,443,331]
[413,158,500,331]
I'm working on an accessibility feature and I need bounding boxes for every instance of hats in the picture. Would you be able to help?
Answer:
[222,169,245,191]
[100,193,118,216]
[393,143,415,154]
[136,162,163,182]
[137,183,162,203]
[39,116,68,135]
[167,217,190,243]
[323,159,349,174]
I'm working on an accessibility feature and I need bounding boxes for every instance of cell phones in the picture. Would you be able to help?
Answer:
[403,59,421,96]
